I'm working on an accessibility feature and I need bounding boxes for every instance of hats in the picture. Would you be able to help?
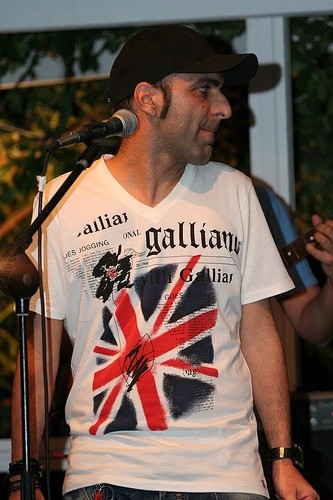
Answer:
[107,24,260,113]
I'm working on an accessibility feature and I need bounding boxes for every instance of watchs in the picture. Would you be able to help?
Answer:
[269,444,303,467]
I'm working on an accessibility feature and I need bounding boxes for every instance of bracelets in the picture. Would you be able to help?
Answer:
[8,457,40,491]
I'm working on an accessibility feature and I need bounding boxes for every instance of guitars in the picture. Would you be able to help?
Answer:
[276,217,330,268]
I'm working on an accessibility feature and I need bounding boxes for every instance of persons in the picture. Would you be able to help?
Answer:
[208,132,333,500]
[7,24,320,500]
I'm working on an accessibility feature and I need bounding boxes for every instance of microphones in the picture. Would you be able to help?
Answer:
[45,109,139,154]
